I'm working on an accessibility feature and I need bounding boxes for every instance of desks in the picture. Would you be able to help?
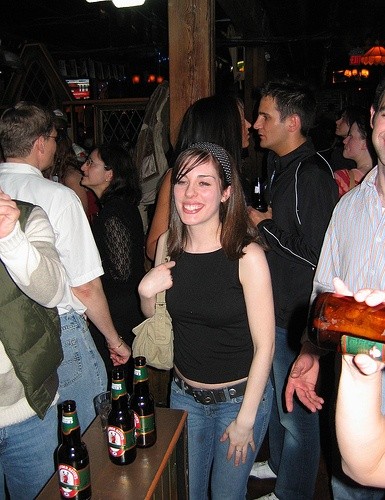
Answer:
[145,362,172,409]
[29,404,188,500]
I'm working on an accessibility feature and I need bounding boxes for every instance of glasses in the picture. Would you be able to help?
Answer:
[86,159,94,166]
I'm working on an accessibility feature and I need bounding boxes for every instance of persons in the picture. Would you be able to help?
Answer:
[284,78,385,500]
[0,190,77,500]
[0,99,130,447]
[327,273,384,492]
[138,142,280,500]
[23,61,385,389]
[145,93,249,273]
[245,73,336,499]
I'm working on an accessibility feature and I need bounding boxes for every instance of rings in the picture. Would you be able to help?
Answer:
[236,449,242,452]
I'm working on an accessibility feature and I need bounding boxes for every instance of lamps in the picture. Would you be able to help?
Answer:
[347,40,385,66]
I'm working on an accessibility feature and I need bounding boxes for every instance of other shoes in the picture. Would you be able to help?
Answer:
[253,492,281,500]
[248,461,277,478]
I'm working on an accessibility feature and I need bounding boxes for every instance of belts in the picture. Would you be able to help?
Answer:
[173,365,247,405]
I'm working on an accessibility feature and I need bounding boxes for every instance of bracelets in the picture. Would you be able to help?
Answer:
[104,336,124,350]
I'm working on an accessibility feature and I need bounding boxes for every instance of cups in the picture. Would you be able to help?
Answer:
[93,390,111,433]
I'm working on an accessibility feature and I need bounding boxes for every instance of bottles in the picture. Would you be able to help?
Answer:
[251,176,268,213]
[106,366,137,465]
[308,295,385,363]
[129,356,157,449]
[55,399,92,500]
